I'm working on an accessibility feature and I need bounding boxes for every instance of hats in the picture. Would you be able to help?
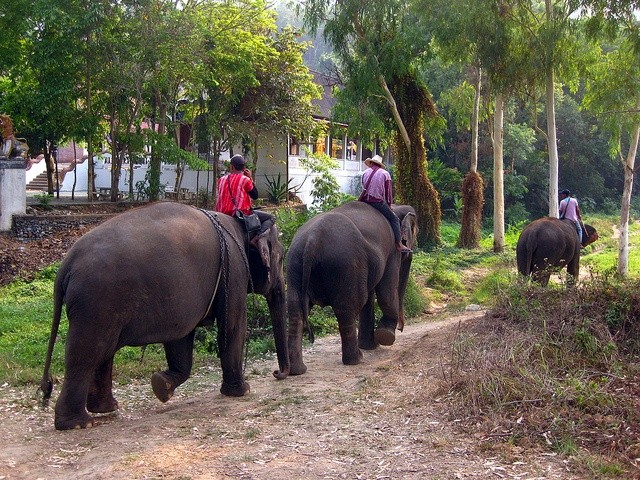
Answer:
[365,155,385,168]
[559,190,569,194]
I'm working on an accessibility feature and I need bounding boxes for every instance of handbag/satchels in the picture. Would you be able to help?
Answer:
[235,209,262,232]
[560,215,565,220]
[358,189,367,201]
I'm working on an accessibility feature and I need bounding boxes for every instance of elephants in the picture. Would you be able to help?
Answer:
[41,200,290,430]
[286,200,419,375]
[517,217,598,288]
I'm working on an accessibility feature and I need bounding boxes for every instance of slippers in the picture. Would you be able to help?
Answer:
[400,247,413,252]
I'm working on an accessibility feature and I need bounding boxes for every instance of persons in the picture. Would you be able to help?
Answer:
[215,154,276,251]
[360,155,411,252]
[559,189,585,249]
